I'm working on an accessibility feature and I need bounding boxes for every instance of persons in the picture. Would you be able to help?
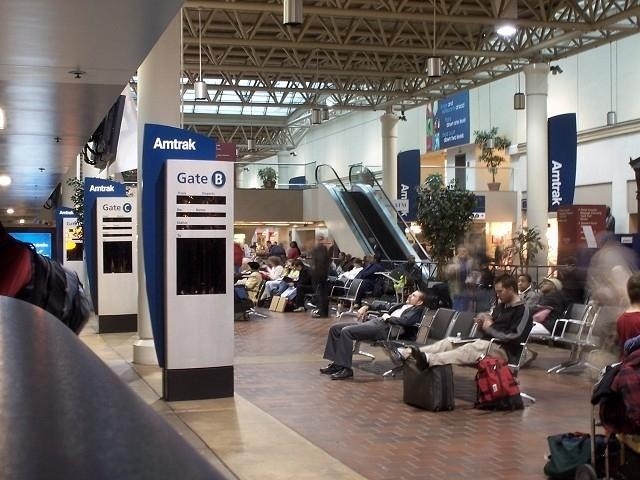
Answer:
[319,289,429,380]
[0,221,32,298]
[382,273,534,375]
[606,205,615,234]
[1,295,233,480]
[234,234,424,318]
[443,230,639,438]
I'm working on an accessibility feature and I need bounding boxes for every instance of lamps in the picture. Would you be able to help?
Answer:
[513,60,526,111]
[606,43,617,127]
[282,0,305,27]
[312,51,322,125]
[248,104,256,151]
[549,64,563,75]
[426,1,443,78]
[194,11,207,101]
[485,72,497,151]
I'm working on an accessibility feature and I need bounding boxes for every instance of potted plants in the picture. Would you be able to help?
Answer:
[258,167,279,190]
[473,126,514,191]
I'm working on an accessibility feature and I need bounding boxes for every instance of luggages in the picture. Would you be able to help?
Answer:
[403,356,455,412]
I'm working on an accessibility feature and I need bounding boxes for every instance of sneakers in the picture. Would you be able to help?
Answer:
[383,343,403,366]
[411,348,427,371]
[307,303,317,308]
[293,307,305,312]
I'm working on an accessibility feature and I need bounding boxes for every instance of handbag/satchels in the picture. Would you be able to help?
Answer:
[544,431,621,479]
[533,304,553,322]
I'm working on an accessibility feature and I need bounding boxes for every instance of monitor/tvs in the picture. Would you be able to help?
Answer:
[83,94,127,170]
[43,184,62,212]
[7,232,52,260]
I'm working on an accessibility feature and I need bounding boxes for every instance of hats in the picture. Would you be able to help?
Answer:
[543,277,563,292]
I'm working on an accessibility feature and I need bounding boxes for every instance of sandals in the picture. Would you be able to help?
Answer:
[265,296,272,301]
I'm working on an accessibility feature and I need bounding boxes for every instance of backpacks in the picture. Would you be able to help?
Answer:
[474,356,524,411]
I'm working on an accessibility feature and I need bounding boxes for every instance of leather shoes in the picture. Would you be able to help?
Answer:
[320,363,343,374]
[331,369,353,380]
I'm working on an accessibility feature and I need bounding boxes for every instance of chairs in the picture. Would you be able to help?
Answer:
[247,278,270,319]
